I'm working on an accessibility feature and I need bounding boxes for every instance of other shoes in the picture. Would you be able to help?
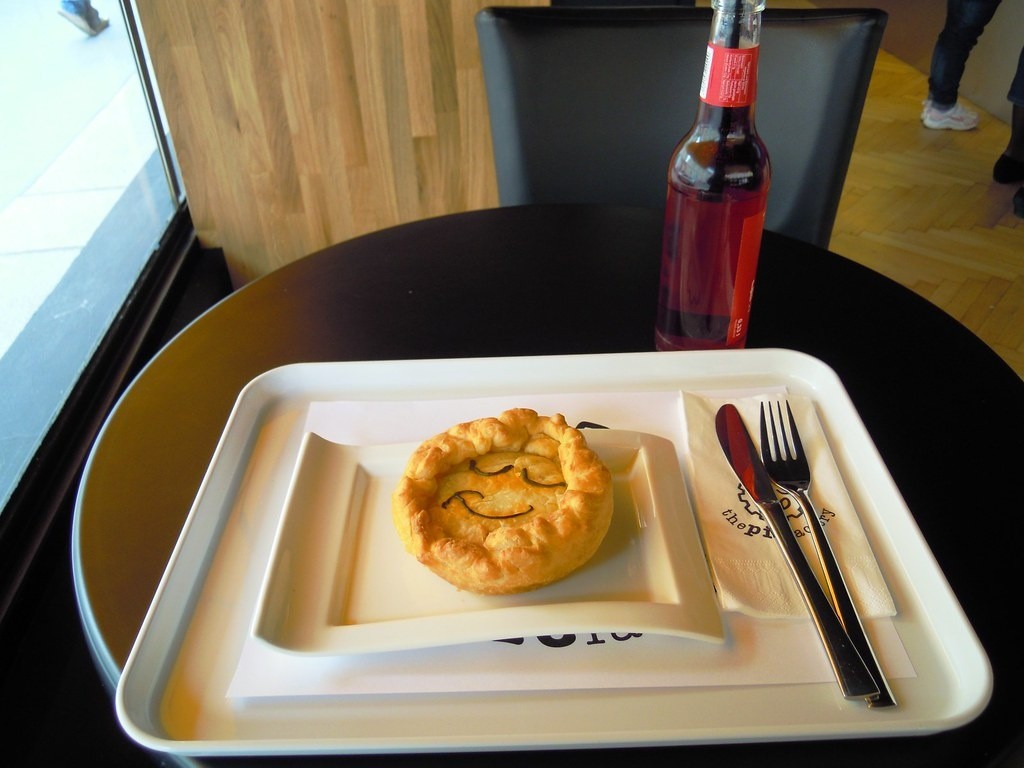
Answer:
[1012,186,1024,217]
[993,154,1024,183]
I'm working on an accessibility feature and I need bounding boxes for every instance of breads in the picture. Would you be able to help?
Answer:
[392,407,614,594]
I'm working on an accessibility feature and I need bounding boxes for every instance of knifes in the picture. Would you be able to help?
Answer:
[714,403,880,701]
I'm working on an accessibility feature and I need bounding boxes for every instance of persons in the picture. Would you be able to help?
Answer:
[920,0,1003,130]
[993,45,1024,218]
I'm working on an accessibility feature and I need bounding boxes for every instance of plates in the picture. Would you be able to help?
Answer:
[249,429,727,657]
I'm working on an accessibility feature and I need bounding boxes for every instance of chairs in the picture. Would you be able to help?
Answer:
[473,8,888,247]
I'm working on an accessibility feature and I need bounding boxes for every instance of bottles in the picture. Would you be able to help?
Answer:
[653,0,773,353]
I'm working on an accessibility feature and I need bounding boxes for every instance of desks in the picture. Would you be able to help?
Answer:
[71,202,1024,767]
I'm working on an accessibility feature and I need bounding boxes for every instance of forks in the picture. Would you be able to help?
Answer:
[760,399,899,709]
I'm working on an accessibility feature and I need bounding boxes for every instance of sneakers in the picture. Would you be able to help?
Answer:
[921,101,978,130]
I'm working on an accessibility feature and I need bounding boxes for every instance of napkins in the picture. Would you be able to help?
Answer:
[684,392,896,619]
